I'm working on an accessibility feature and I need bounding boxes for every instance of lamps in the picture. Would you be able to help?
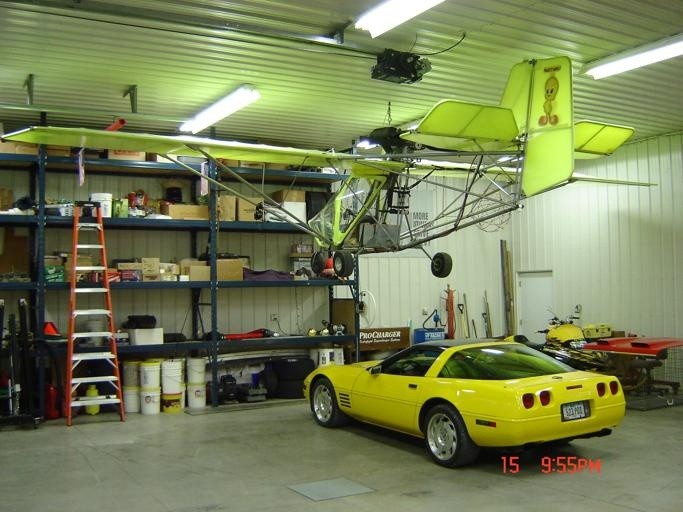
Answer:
[354,0,449,39]
[582,33,683,81]
[179,82,261,134]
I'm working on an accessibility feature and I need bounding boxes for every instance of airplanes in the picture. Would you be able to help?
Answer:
[0,56,662,279]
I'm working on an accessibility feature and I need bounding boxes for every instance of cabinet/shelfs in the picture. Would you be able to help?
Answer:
[0,151,363,422]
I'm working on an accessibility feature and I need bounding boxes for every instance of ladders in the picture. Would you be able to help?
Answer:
[66,200,126,426]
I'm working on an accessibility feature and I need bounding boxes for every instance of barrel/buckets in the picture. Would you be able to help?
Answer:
[45,384,60,419]
[85,315,106,346]
[60,383,78,417]
[90,192,113,218]
[122,356,206,413]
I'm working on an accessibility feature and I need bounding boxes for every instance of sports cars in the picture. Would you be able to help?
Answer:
[301,336,630,470]
